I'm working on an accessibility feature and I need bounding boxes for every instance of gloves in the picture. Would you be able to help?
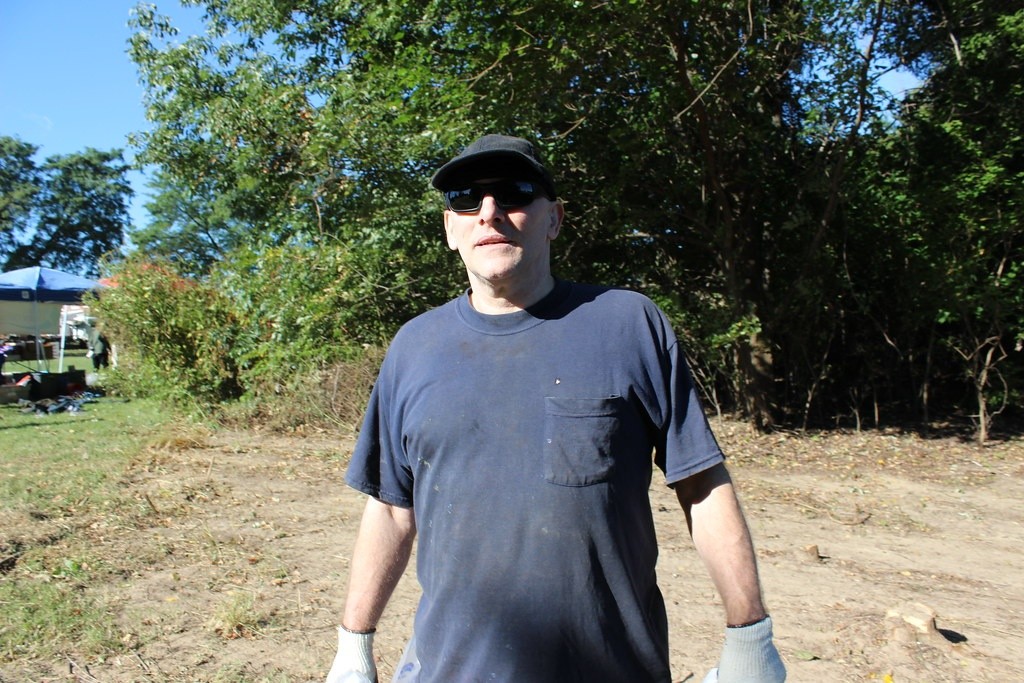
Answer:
[325,623,378,683]
[717,614,786,683]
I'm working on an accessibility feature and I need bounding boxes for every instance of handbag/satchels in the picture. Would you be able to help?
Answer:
[85,350,94,359]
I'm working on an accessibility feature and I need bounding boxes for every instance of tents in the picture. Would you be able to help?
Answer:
[0,265,118,378]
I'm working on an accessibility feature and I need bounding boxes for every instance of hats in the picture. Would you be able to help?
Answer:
[433,134,553,204]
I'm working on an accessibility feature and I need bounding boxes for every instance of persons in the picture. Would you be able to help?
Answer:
[88,323,114,373]
[325,133,786,683]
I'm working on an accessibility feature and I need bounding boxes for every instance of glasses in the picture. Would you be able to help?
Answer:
[443,171,553,214]
